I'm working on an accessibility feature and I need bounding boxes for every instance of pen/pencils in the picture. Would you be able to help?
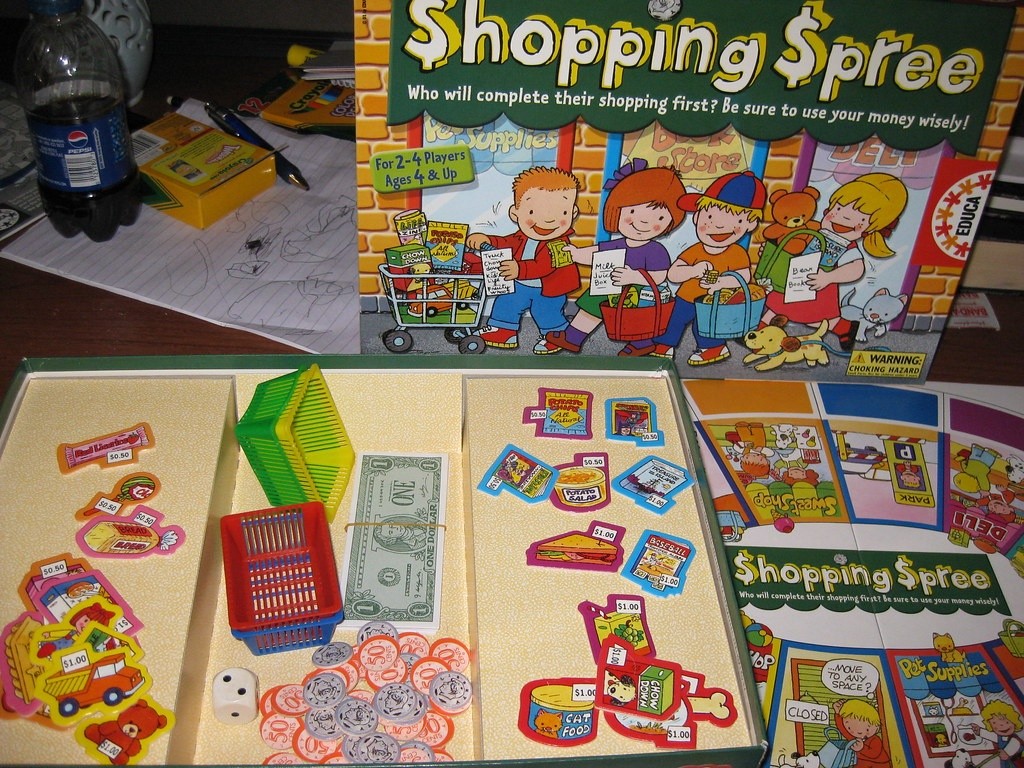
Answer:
[202,99,311,191]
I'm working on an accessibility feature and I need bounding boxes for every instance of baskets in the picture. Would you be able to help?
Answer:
[235,362,355,527]
[221,503,345,657]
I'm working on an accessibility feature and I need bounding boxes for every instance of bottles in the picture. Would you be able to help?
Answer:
[16,0,142,243]
